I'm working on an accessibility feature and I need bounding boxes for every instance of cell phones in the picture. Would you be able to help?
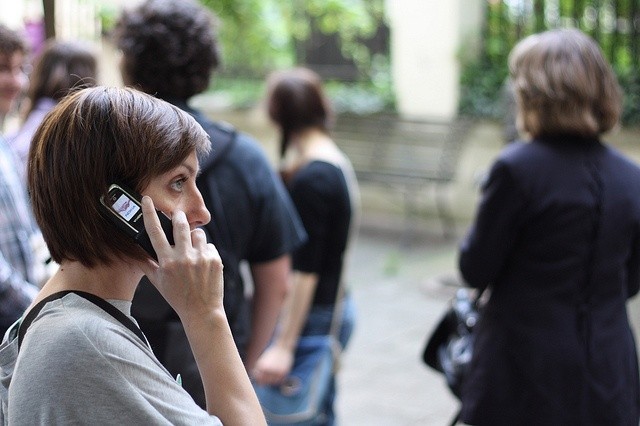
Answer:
[96,180,175,263]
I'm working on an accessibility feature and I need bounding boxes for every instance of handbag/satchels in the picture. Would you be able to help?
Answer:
[252,334,345,425]
[421,291,531,423]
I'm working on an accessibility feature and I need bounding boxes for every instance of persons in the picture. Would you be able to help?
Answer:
[1,22,62,346]
[0,84,269,426]
[245,64,362,426]
[448,24,639,426]
[108,0,314,415]
[6,35,98,185]
